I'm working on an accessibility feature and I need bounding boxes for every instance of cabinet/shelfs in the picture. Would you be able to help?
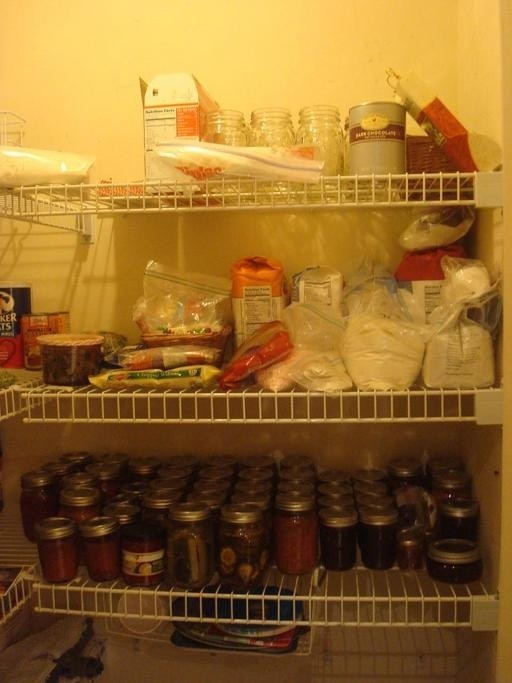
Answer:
[0,173,511,683]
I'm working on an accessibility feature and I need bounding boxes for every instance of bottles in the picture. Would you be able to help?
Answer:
[19,451,479,588]
[203,105,344,188]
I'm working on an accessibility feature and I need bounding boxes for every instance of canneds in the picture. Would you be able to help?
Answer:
[0,280,30,372]
[20,451,483,590]
[345,100,407,203]
[18,312,71,369]
